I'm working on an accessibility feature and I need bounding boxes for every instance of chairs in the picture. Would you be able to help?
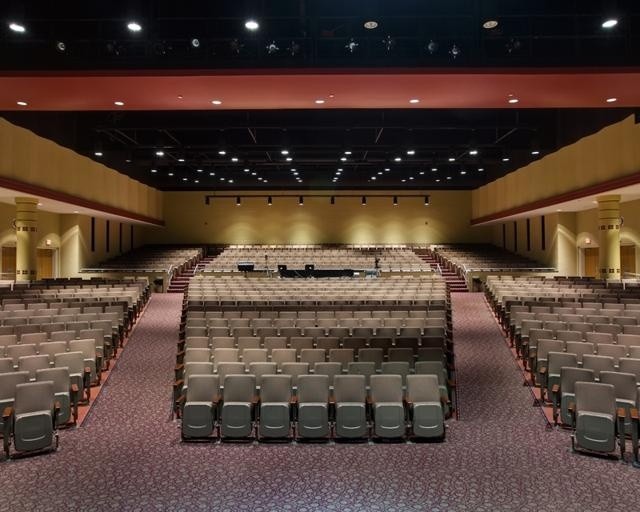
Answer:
[481,277,639,471]
[172,244,458,448]
[86,247,172,292]
[459,245,557,291]
[3,274,150,460]
